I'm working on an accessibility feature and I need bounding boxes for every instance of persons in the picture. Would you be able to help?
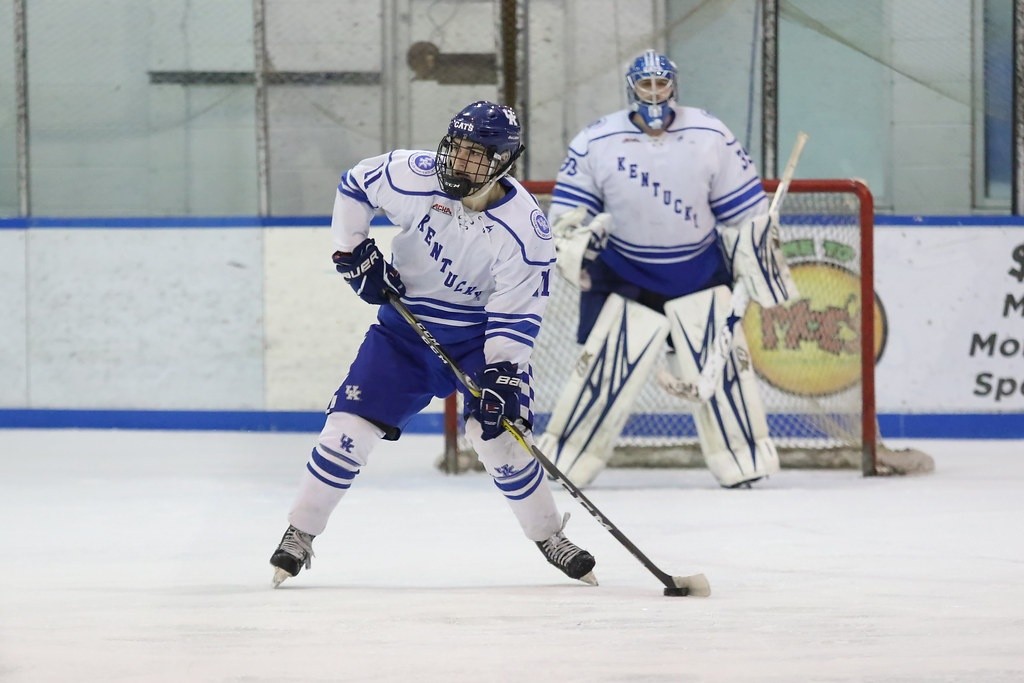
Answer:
[535,50,789,490]
[268,100,600,587]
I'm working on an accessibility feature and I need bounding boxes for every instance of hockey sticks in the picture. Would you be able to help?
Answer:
[656,132,809,400]
[385,288,711,598]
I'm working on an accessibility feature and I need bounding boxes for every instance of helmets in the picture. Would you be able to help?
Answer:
[435,101,525,200]
[625,48,677,130]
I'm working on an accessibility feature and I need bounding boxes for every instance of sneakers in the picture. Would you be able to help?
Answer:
[534,512,598,586]
[270,524,316,588]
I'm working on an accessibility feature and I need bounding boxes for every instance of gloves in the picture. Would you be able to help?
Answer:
[332,237,405,305]
[466,361,520,441]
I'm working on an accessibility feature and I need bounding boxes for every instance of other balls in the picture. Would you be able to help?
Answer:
[663,587,689,597]
